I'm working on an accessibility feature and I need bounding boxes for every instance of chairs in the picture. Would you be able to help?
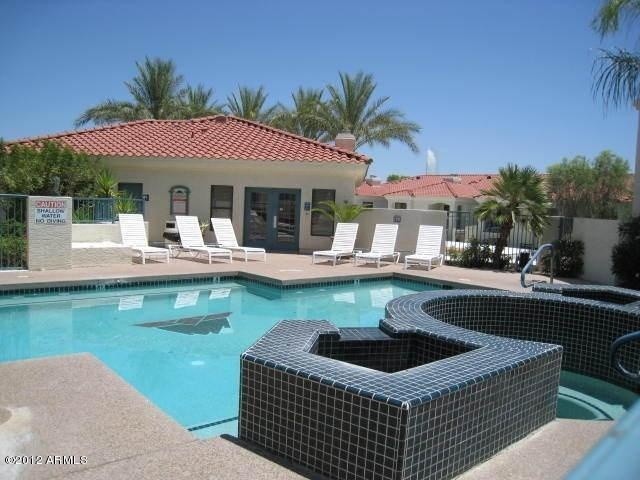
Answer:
[370,287,393,308]
[357,224,400,269]
[312,223,360,266]
[174,291,200,308]
[208,288,231,300]
[333,292,355,304]
[168,216,232,264]
[404,224,444,271]
[119,294,144,311]
[211,218,266,262]
[119,213,169,265]
